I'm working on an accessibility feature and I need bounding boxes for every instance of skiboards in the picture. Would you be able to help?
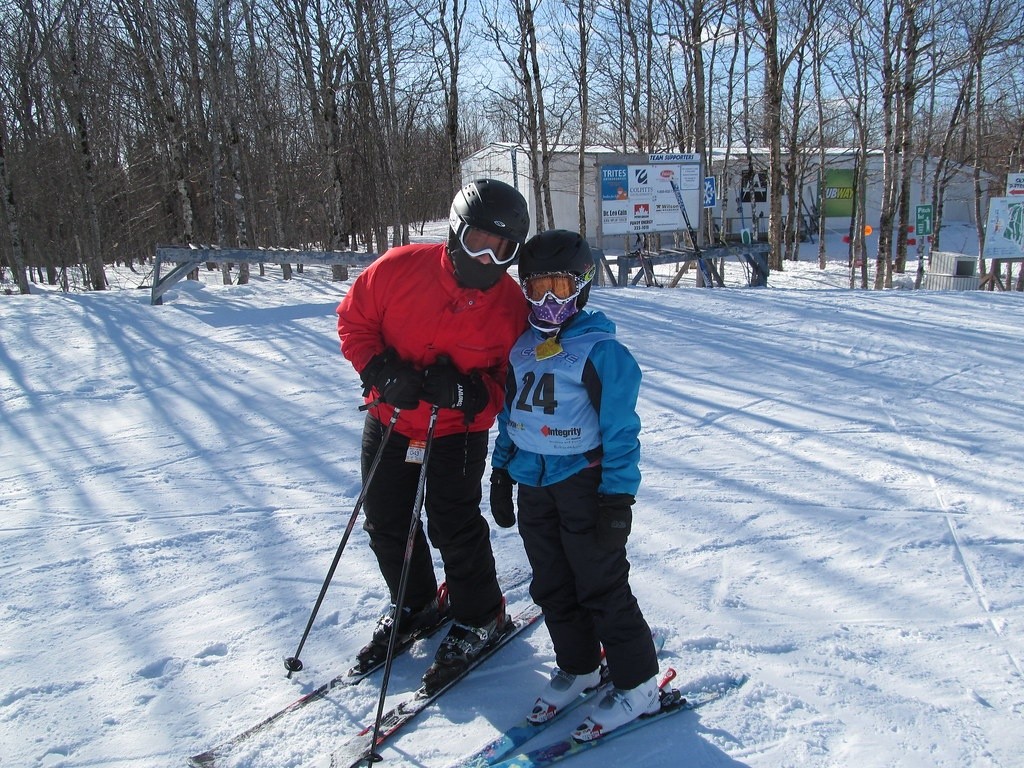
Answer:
[185,560,544,768]
[444,632,746,767]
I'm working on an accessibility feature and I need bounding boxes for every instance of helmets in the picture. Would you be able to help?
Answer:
[446,179,531,289]
[517,228,597,328]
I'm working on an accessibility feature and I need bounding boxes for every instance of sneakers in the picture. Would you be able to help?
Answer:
[434,612,500,664]
[540,666,601,710]
[373,595,440,643]
[588,676,659,729]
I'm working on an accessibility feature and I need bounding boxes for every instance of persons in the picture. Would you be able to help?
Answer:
[490,230,660,743]
[337,179,530,686]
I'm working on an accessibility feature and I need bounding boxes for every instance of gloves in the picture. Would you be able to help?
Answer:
[597,493,636,551]
[419,354,489,422]
[360,349,419,410]
[490,468,517,528]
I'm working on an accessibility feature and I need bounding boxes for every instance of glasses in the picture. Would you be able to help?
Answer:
[521,270,580,307]
[456,221,520,266]
